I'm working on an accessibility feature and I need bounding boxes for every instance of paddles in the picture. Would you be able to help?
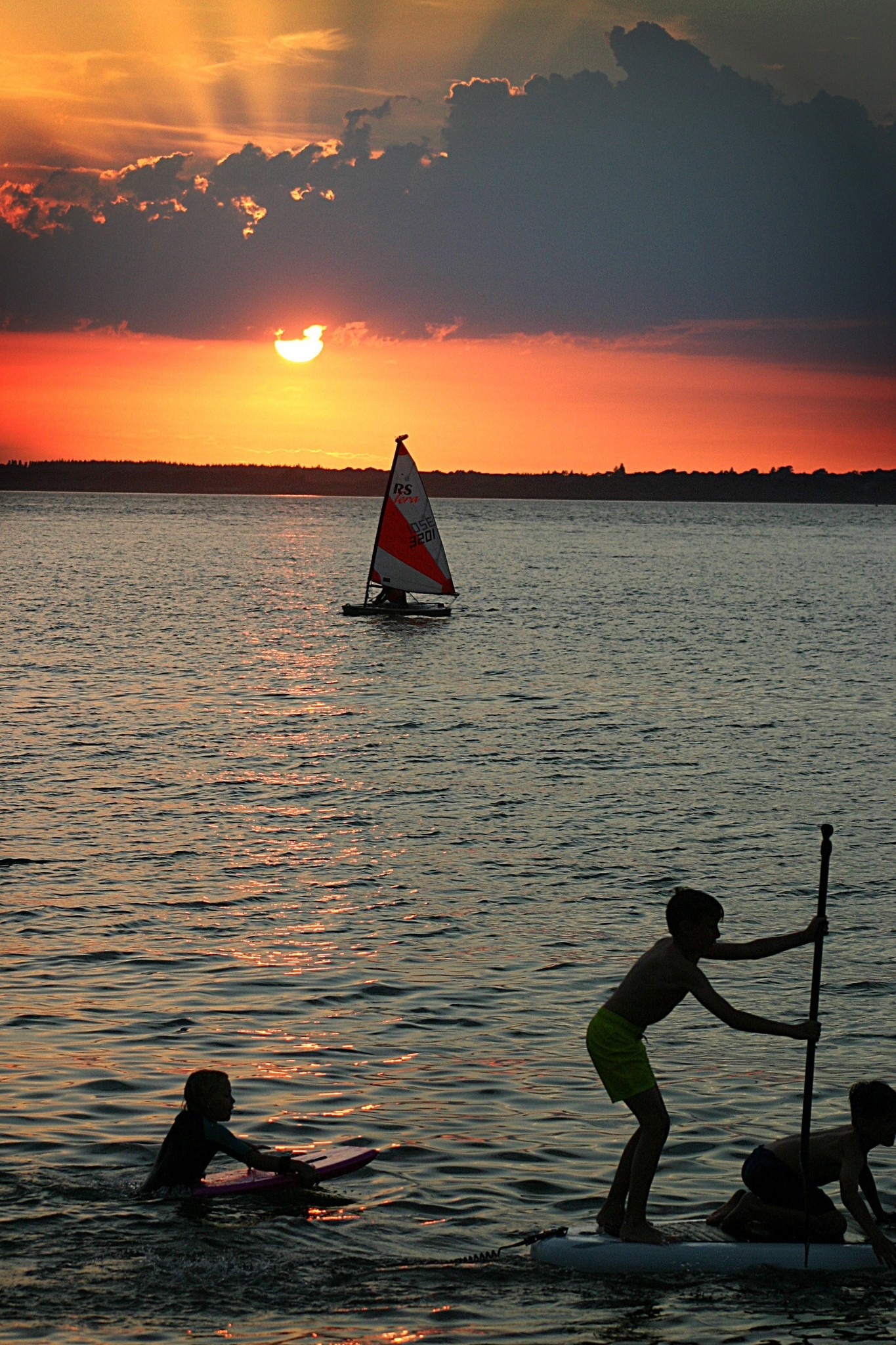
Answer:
[798,823,835,1131]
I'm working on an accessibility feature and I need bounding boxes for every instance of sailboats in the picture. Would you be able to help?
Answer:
[341,434,461,616]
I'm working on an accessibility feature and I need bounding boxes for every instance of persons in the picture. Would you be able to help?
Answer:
[373,586,410,608]
[585,886,830,1244]
[140,1068,318,1204]
[706,1078,896,1275]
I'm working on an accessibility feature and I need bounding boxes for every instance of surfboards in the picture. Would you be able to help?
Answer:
[531,1219,896,1284]
[195,1146,378,1195]
